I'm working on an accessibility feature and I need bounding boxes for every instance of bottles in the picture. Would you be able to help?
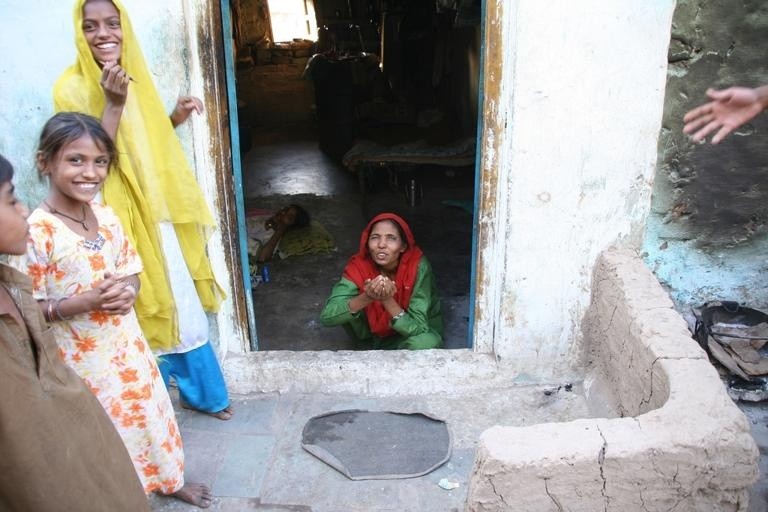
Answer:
[404,179,423,208]
[262,264,272,285]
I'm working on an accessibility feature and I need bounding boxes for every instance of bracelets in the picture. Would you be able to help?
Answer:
[125,281,139,295]
[54,297,72,321]
[46,298,55,323]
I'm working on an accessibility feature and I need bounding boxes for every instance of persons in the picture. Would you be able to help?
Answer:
[8,110,216,510]
[1,150,159,512]
[49,1,237,424]
[243,203,310,265]
[318,211,446,353]
[679,82,766,149]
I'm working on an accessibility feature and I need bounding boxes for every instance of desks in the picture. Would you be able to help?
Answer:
[346,125,477,218]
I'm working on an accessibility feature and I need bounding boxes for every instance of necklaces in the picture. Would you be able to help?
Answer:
[42,197,91,234]
[266,220,273,228]
